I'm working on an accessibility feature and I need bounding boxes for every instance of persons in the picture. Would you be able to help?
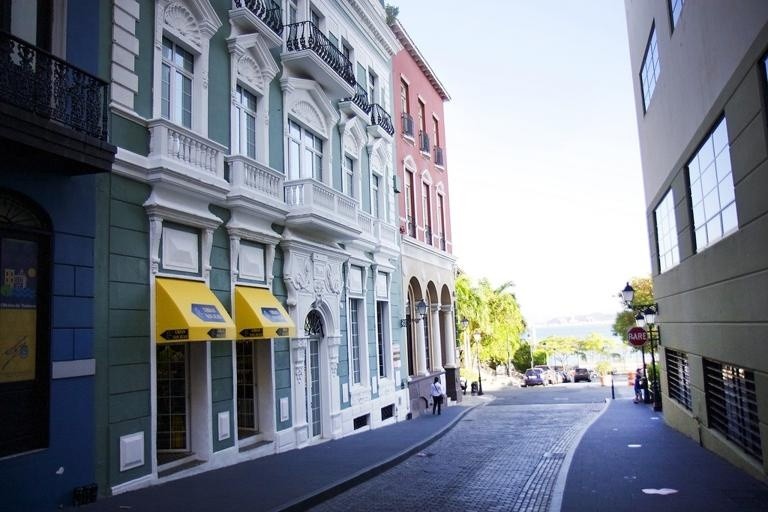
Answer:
[634,376,645,402]
[430,377,445,416]
[635,367,643,377]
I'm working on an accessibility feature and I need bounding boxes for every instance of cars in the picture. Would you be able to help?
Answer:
[523,364,597,385]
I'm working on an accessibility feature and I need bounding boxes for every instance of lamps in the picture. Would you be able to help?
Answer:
[411,299,429,323]
[621,282,658,313]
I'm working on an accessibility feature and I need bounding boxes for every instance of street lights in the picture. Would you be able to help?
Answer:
[529,342,545,367]
[634,313,650,404]
[644,307,661,411]
[471,329,484,394]
